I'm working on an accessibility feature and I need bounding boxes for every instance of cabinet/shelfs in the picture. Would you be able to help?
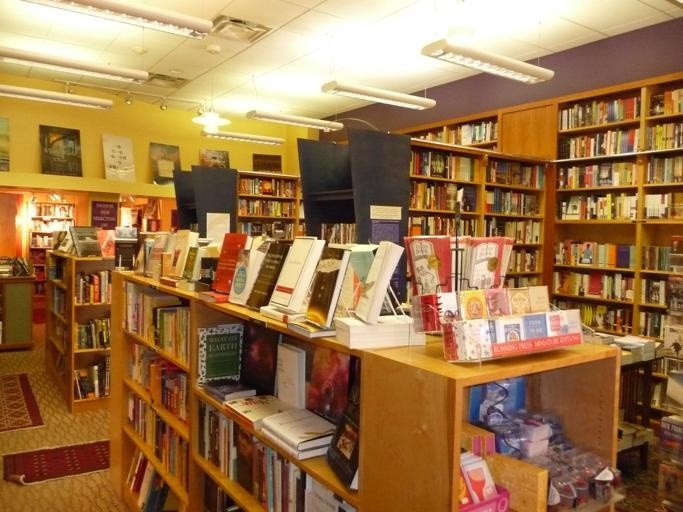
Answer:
[409,117,497,150]
[107,271,621,512]
[31,202,75,321]
[238,172,306,240]
[45,249,115,413]
[408,139,551,289]
[551,75,683,436]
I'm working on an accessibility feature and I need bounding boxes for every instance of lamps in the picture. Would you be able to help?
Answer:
[24,0,213,41]
[0,29,231,133]
[201,130,286,147]
[246,60,344,131]
[422,1,554,84]
[321,41,435,112]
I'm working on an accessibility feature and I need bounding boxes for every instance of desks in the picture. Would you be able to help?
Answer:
[0,273,36,352]
[210,122,212,124]
[598,337,657,470]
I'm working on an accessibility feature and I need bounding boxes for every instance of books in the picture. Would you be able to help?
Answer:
[416,119,498,145]
[239,176,306,239]
[559,84,683,459]
[123,224,426,512]
[48,225,115,400]
[410,148,544,287]
